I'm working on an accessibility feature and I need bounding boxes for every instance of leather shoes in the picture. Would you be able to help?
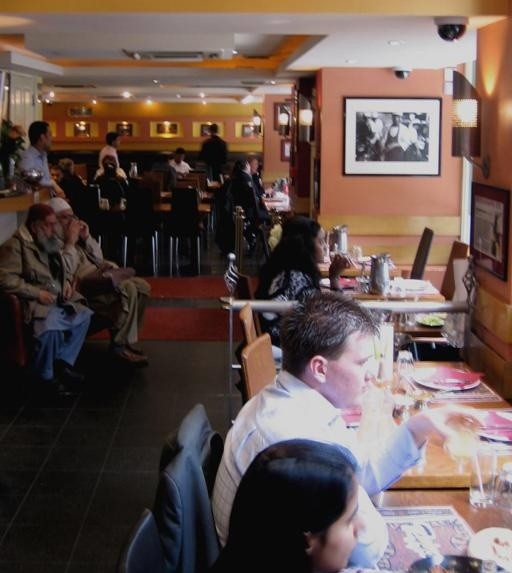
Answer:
[42,376,73,402]
[54,364,77,390]
[110,342,148,366]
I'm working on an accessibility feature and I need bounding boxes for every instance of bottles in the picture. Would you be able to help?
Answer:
[270,177,288,198]
[23,167,45,184]
[493,461,511,510]
[354,263,370,295]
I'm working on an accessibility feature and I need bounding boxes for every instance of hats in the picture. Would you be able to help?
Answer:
[48,197,71,214]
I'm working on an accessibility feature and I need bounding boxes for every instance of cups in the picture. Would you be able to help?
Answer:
[392,276,404,298]
[395,350,413,392]
[468,443,497,509]
[321,245,330,262]
[353,245,363,260]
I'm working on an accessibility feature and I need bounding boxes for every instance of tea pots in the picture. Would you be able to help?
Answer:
[130,162,138,177]
[369,253,392,297]
[328,223,349,255]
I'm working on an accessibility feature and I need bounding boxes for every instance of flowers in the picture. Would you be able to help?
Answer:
[0,118,25,175]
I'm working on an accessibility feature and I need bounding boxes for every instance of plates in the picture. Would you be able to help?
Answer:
[474,408,512,442]
[412,527,511,572]
[396,280,428,291]
[413,312,444,327]
[415,366,485,391]
[357,256,371,262]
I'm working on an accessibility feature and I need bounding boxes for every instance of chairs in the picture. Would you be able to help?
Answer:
[440,240,470,300]
[410,227,434,280]
[398,321,451,360]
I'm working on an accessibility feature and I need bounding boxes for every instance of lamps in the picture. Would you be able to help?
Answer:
[278,104,291,137]
[253,109,263,135]
[296,90,316,144]
[451,70,491,178]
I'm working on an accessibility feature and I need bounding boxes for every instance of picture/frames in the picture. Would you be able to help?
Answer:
[273,102,296,132]
[342,95,442,178]
[280,138,292,162]
[469,182,512,283]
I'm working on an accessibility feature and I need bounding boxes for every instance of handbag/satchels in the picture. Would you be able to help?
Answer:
[84,267,135,292]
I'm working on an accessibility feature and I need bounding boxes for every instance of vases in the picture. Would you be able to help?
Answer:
[0,157,16,187]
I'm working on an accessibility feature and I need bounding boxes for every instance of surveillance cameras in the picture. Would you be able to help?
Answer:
[392,66,412,81]
[434,17,470,42]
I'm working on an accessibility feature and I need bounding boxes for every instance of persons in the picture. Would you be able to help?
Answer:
[207,439,367,572]
[0,201,92,403]
[47,195,150,363]
[355,112,430,161]
[97,131,122,169]
[197,123,229,167]
[48,154,272,251]
[12,119,67,230]
[252,213,355,350]
[212,292,487,568]
